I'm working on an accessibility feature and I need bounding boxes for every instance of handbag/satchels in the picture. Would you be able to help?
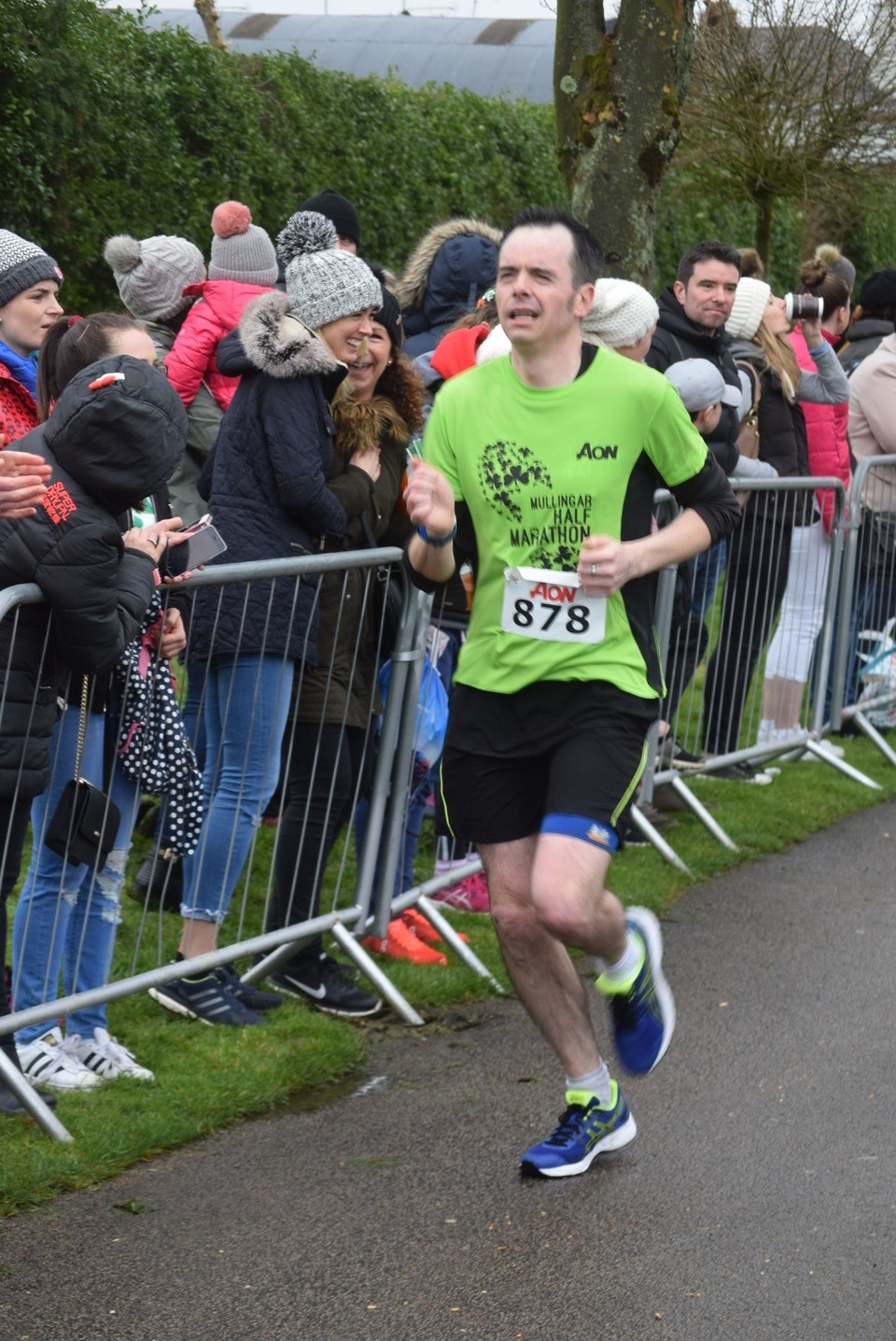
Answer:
[44,777,121,872]
[858,618,896,727]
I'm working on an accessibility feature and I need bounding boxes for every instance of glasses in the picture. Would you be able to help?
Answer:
[152,364,168,377]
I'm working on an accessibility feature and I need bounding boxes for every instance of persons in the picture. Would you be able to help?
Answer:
[102,189,896,1024]
[398,214,743,1179]
[15,313,187,1092]
[0,226,204,1116]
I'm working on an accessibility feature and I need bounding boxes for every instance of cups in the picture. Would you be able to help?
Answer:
[784,292,824,320]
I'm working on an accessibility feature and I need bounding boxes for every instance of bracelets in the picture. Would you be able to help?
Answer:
[417,525,457,547]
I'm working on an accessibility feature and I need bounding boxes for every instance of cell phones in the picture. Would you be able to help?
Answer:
[154,526,227,585]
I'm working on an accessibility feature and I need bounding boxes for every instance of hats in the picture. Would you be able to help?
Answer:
[369,264,401,349]
[814,243,856,292]
[103,187,383,328]
[0,229,65,308]
[664,357,741,412]
[580,278,660,348]
[724,277,771,340]
[859,268,896,310]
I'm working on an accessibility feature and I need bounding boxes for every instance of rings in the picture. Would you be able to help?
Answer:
[592,565,596,575]
[147,539,157,546]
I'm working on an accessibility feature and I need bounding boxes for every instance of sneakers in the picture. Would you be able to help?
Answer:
[622,784,716,846]
[595,903,677,1077]
[391,905,471,944]
[360,918,447,966]
[654,723,845,784]
[519,1077,637,1177]
[0,1026,155,1112]
[433,873,491,913]
[147,948,384,1027]
